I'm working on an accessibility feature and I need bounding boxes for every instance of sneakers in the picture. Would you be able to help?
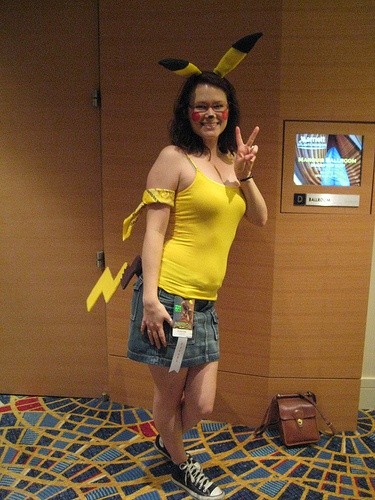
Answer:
[154,433,191,462]
[171,455,225,500]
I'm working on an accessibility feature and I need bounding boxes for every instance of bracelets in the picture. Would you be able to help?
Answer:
[238,174,253,182]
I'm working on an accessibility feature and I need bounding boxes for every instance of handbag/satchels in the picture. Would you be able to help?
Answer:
[276,390,321,447]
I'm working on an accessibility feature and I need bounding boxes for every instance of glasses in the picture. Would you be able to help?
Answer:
[188,102,228,112]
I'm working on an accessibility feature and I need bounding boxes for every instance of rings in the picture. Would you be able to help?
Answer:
[147,330,152,333]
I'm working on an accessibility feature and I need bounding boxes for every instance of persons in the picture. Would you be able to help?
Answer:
[87,31,268,500]
